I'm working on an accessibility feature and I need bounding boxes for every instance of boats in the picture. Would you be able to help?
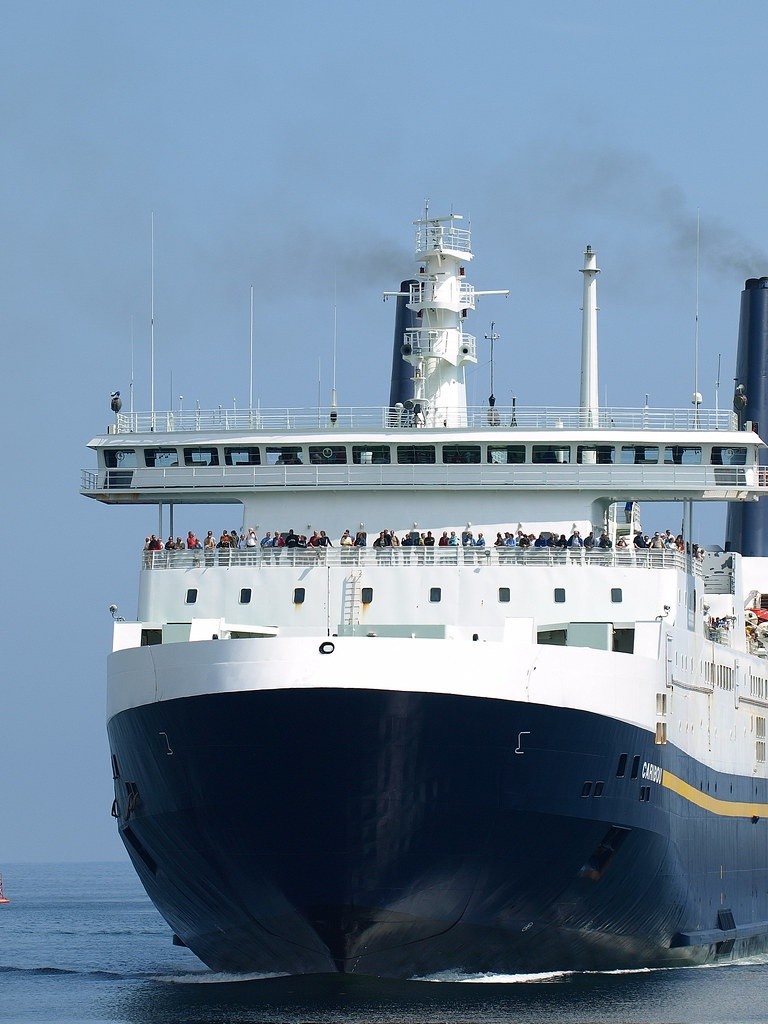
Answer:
[749,607,768,624]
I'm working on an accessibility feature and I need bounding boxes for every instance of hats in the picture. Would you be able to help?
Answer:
[169,536,173,540]
[695,543,700,548]
[504,532,509,534]
[428,531,432,534]
[208,531,212,535]
[177,537,181,542]
[636,530,642,534]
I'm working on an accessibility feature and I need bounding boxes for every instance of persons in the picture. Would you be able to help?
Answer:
[144,529,367,567]
[708,617,764,656]
[373,529,704,565]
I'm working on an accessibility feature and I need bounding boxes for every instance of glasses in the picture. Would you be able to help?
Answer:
[638,533,642,534]
[655,535,660,537]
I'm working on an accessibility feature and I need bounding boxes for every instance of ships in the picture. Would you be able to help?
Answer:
[72,205,768,982]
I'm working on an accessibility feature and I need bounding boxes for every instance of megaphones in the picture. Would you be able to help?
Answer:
[400,344,412,355]
